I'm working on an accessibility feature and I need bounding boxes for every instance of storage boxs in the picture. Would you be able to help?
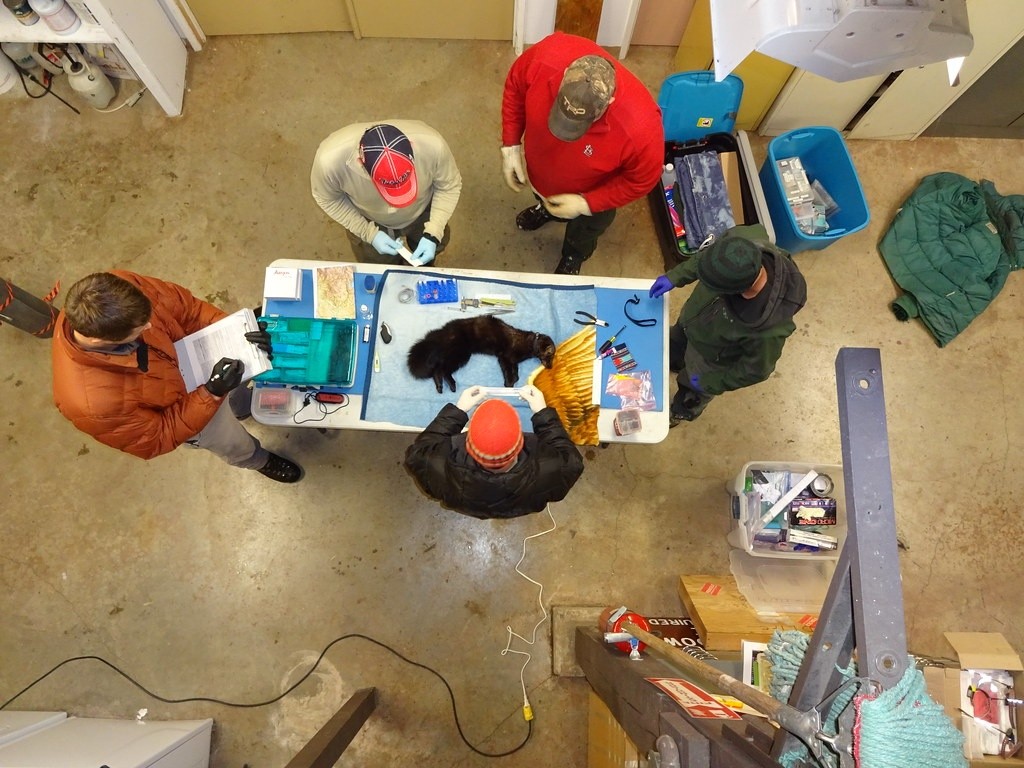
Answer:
[758,125,870,255]
[725,460,848,561]
[252,388,296,419]
[922,631,1024,768]
[250,317,359,388]
[647,70,776,272]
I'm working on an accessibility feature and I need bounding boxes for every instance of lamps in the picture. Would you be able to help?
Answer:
[708,0,974,88]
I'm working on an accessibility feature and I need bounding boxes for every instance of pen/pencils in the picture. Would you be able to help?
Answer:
[211,362,231,382]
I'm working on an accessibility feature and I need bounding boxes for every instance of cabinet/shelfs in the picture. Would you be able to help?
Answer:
[0,0,187,116]
[674,0,1024,138]
[0,710,214,768]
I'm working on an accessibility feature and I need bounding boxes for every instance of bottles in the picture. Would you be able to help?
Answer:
[2,42,38,69]
[662,163,676,189]
[359,304,372,321]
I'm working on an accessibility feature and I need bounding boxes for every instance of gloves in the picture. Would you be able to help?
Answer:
[371,231,404,256]
[518,384,547,414]
[690,375,704,392]
[411,237,436,266]
[500,144,527,191]
[543,194,593,219]
[455,384,489,413]
[650,276,674,301]
[204,357,244,396]
[245,321,274,361]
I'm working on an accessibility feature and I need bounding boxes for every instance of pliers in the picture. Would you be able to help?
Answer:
[573,311,609,328]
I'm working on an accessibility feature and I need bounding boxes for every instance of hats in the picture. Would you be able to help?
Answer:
[549,55,616,142]
[358,124,418,208]
[465,398,523,470]
[696,237,761,292]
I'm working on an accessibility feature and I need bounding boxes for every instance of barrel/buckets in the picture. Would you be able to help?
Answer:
[28,0,81,35]
[0,51,17,93]
[2,0,38,25]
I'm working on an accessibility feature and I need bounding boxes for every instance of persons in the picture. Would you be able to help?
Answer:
[51,272,305,483]
[405,385,584,519]
[310,119,464,267]
[648,223,807,429]
[502,31,664,274]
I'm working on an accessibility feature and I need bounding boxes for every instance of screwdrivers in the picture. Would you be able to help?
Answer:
[599,325,627,353]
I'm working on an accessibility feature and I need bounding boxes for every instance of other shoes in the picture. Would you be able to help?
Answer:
[516,203,569,231]
[256,453,301,482]
[552,256,581,276]
[667,403,682,429]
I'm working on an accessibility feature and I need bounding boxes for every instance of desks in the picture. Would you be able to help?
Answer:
[250,258,671,447]
